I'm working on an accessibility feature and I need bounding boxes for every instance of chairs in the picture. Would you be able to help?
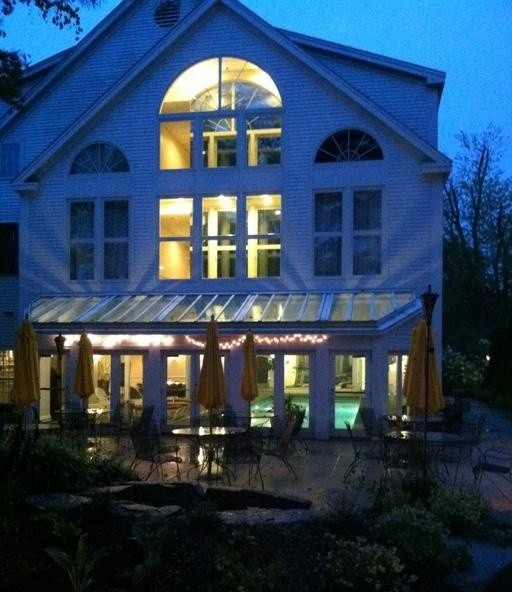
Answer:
[343,404,512,503]
[52,403,311,490]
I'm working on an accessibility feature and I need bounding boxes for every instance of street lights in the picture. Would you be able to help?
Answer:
[54,330,65,448]
[419,284,439,485]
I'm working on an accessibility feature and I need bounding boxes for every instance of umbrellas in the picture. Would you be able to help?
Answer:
[13,312,40,439]
[196,314,228,481]
[396,318,446,463]
[240,327,259,456]
[72,328,94,412]
